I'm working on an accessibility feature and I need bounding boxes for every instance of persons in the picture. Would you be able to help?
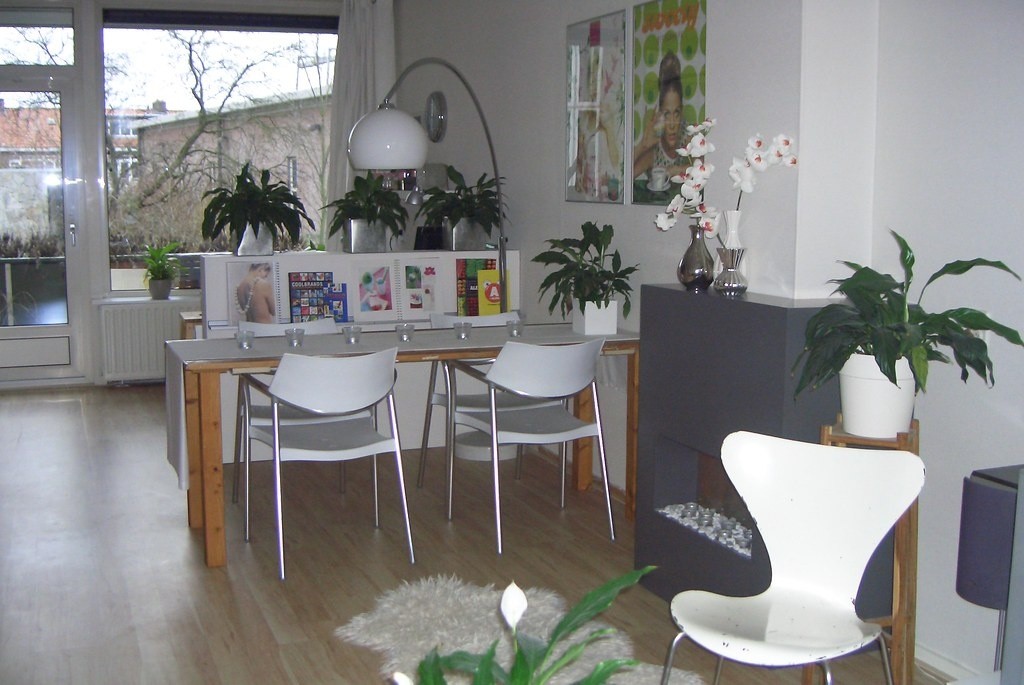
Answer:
[633,52,704,182]
[238,263,276,324]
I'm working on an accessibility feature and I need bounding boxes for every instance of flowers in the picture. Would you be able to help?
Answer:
[729,131,798,210]
[656,118,716,239]
[391,560,663,684]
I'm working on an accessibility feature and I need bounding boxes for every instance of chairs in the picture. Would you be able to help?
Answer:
[231,346,417,581]
[415,338,616,555]
[658,429,926,685]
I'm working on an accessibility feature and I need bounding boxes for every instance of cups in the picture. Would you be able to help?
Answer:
[453,322,472,343]
[506,320,524,338]
[342,327,362,345]
[395,324,415,344]
[285,328,305,349]
[233,330,255,350]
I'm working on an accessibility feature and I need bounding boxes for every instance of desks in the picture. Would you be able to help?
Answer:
[162,319,642,567]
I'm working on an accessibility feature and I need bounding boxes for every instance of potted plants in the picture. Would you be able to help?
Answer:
[791,228,1024,442]
[412,166,509,250]
[528,221,639,337]
[197,159,315,260]
[140,239,187,301]
[317,169,411,252]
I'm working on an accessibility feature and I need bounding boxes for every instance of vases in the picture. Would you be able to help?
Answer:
[676,225,714,293]
[713,209,745,281]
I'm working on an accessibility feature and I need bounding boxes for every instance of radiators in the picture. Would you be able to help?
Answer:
[88,294,197,388]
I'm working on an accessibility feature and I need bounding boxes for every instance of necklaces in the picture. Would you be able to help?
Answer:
[234,276,261,314]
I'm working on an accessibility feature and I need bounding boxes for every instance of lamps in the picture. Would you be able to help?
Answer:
[346,57,508,315]
